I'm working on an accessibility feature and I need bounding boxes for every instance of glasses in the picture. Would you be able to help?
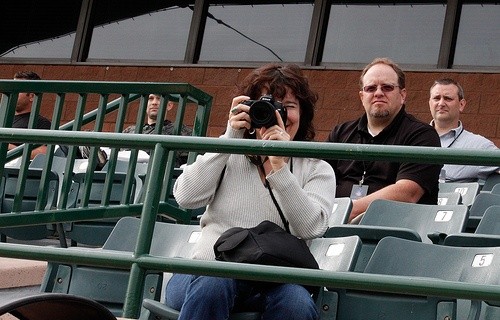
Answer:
[363,83,402,93]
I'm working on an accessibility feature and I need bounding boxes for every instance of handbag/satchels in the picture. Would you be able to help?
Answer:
[212,219,322,314]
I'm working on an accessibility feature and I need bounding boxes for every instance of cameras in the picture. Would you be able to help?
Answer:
[236,95,287,129]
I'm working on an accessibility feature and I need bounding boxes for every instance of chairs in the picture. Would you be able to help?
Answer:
[0,144,500,320]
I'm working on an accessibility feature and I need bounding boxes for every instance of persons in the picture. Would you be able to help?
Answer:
[428,78,500,184]
[7,71,51,160]
[323,57,444,225]
[119,93,193,168]
[164,62,337,320]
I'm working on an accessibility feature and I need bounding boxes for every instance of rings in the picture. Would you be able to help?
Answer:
[231,107,238,115]
